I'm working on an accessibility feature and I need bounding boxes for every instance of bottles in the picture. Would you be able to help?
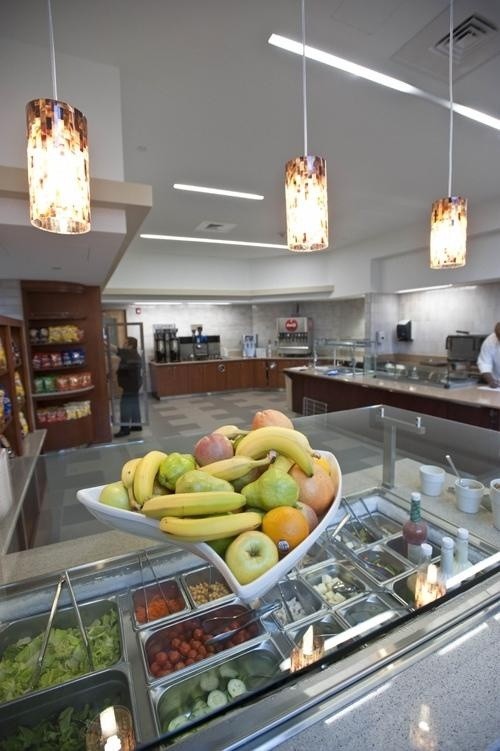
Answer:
[403,491,429,563]
[432,528,478,591]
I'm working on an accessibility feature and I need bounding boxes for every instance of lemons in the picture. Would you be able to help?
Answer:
[313,457,331,473]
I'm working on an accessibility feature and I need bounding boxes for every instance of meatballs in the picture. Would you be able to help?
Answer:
[149,619,251,679]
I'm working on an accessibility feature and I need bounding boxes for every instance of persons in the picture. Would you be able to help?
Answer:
[476,323,500,388]
[103,337,143,437]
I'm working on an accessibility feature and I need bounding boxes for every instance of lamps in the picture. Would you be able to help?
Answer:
[283,1,331,252]
[23,1,90,234]
[429,0,469,270]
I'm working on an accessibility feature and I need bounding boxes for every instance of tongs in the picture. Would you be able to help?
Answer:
[329,536,393,581]
[136,547,174,626]
[342,496,382,542]
[276,574,317,625]
[199,600,280,647]
[33,568,96,691]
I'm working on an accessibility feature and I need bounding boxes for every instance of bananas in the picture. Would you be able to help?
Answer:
[132,451,167,507]
[155,511,261,543]
[234,426,320,462]
[197,452,277,481]
[120,459,143,509]
[214,425,249,437]
[140,492,246,520]
[234,436,314,478]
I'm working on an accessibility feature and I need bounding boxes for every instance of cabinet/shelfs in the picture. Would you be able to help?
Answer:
[148,356,345,402]
[3,449,48,554]
[23,311,95,451]
[0,314,36,458]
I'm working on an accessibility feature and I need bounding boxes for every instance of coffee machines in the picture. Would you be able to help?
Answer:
[275,316,313,356]
[177,337,222,363]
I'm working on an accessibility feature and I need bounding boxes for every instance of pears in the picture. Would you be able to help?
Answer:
[158,452,196,492]
[174,469,235,495]
[240,468,300,512]
[98,480,130,511]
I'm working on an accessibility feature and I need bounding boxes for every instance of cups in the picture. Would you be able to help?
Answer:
[454,479,487,513]
[489,477,500,531]
[417,464,445,496]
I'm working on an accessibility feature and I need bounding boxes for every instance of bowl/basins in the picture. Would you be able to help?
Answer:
[75,448,343,601]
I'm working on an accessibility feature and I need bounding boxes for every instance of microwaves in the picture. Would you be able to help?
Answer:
[445,334,488,364]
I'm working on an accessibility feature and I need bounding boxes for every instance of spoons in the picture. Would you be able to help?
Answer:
[332,583,397,598]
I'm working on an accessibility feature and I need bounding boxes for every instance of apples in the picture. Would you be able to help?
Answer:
[296,500,319,533]
[251,410,294,433]
[288,461,336,515]
[224,530,279,585]
[193,433,236,468]
